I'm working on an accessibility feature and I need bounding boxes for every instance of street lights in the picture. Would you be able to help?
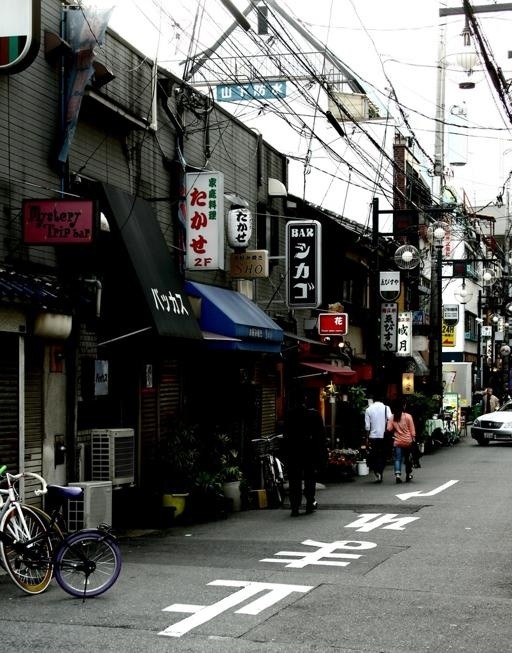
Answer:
[439,255,512,396]
[370,193,420,358]
[425,219,453,413]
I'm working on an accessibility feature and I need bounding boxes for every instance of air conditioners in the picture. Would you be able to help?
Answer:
[91,428,136,484]
[67,481,113,532]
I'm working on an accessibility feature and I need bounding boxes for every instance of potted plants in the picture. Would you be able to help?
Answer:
[224,463,242,511]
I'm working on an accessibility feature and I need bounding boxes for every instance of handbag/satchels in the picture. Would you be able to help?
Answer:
[384,431,393,445]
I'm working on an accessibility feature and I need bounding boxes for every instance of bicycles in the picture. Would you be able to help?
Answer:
[252,433,287,507]
[0,464,123,605]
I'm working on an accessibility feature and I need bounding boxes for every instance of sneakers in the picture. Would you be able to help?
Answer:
[374,472,414,484]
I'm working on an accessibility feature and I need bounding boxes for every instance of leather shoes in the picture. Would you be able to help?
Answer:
[306,501,317,512]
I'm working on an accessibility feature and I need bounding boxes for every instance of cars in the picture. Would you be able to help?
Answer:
[471,398,512,447]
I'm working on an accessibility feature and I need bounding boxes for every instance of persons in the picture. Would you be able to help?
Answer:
[387,397,416,482]
[482,387,500,414]
[283,389,327,517]
[364,391,392,481]
